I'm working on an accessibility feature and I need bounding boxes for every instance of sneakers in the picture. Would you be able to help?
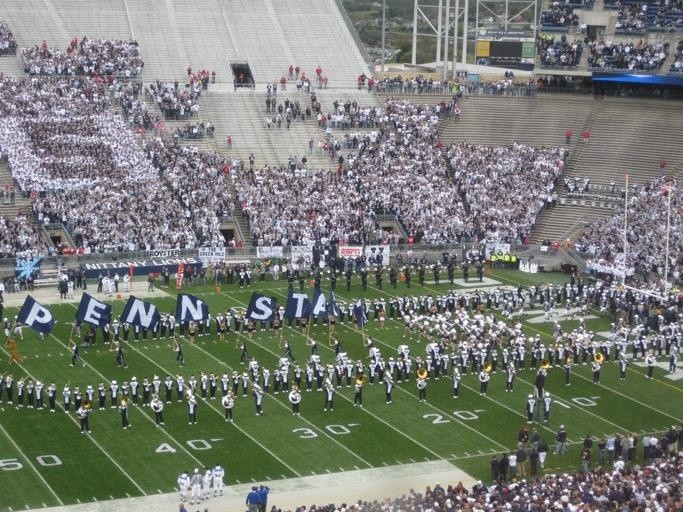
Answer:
[179,492,224,505]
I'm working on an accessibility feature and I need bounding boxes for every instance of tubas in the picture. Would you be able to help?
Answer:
[76,385,302,418]
[321,353,604,391]
[618,346,678,365]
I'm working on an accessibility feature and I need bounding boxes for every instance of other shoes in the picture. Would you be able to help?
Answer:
[403,301,683,403]
[0,380,402,434]
[182,360,184,364]
[83,364,85,368]
[217,317,401,335]
[179,365,182,368]
[293,360,297,363]
[69,364,74,367]
[544,420,547,422]
[124,365,128,368]
[526,421,532,424]
[240,362,244,364]
[104,333,210,345]
[118,365,122,368]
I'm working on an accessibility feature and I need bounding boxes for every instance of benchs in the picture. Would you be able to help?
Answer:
[0,1,683,260]
